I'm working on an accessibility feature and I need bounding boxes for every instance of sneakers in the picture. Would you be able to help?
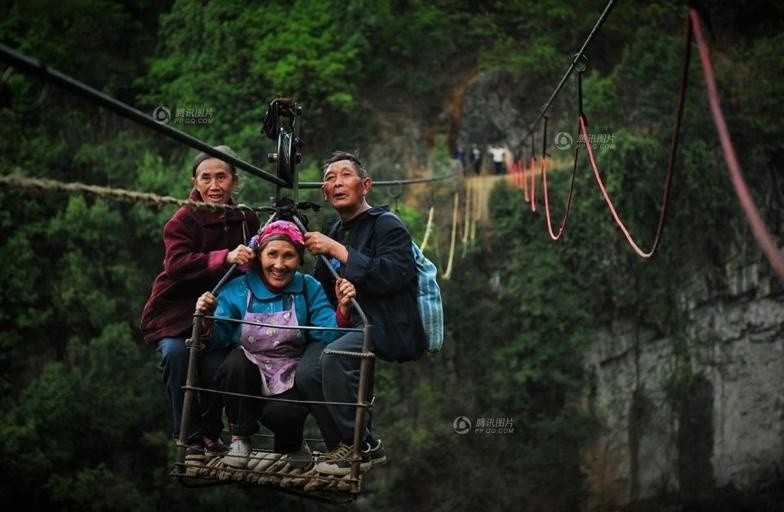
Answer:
[283,438,312,468]
[200,433,226,457]
[371,436,387,468]
[220,438,253,469]
[315,441,372,475]
[182,443,206,461]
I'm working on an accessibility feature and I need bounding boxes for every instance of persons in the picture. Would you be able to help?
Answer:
[139,143,261,462]
[194,219,357,475]
[487,142,506,175]
[468,141,483,175]
[292,149,427,476]
[454,143,467,177]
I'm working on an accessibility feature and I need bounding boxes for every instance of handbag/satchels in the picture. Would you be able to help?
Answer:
[372,211,444,354]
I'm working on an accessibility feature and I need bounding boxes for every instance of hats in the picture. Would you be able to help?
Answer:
[256,220,307,265]
